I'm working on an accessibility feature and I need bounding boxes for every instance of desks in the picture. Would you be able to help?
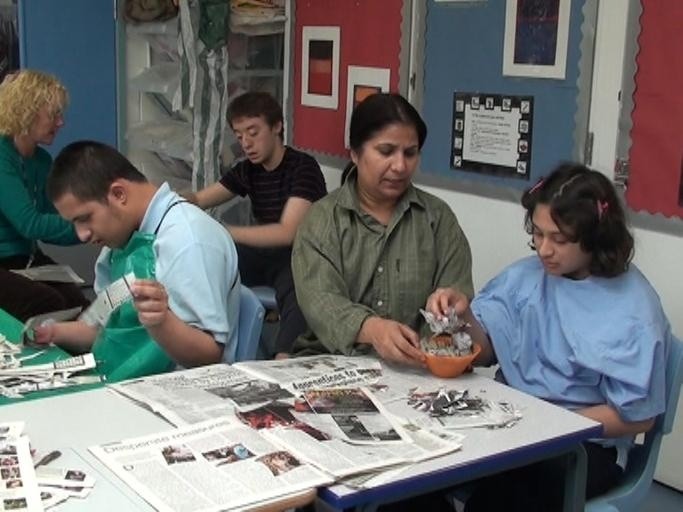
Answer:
[316,353,603,512]
[222,486,319,512]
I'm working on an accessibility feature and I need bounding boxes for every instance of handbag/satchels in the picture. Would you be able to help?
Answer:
[123,0,179,24]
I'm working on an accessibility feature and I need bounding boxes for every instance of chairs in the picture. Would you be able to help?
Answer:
[235,283,266,364]
[585,333,683,512]
[249,285,279,310]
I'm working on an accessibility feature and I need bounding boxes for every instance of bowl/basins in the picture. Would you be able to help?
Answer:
[420,335,482,377]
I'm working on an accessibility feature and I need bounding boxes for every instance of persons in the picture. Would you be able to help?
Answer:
[172,89,330,360]
[421,158,672,511]
[0,67,89,329]
[289,90,476,371]
[30,138,244,389]
